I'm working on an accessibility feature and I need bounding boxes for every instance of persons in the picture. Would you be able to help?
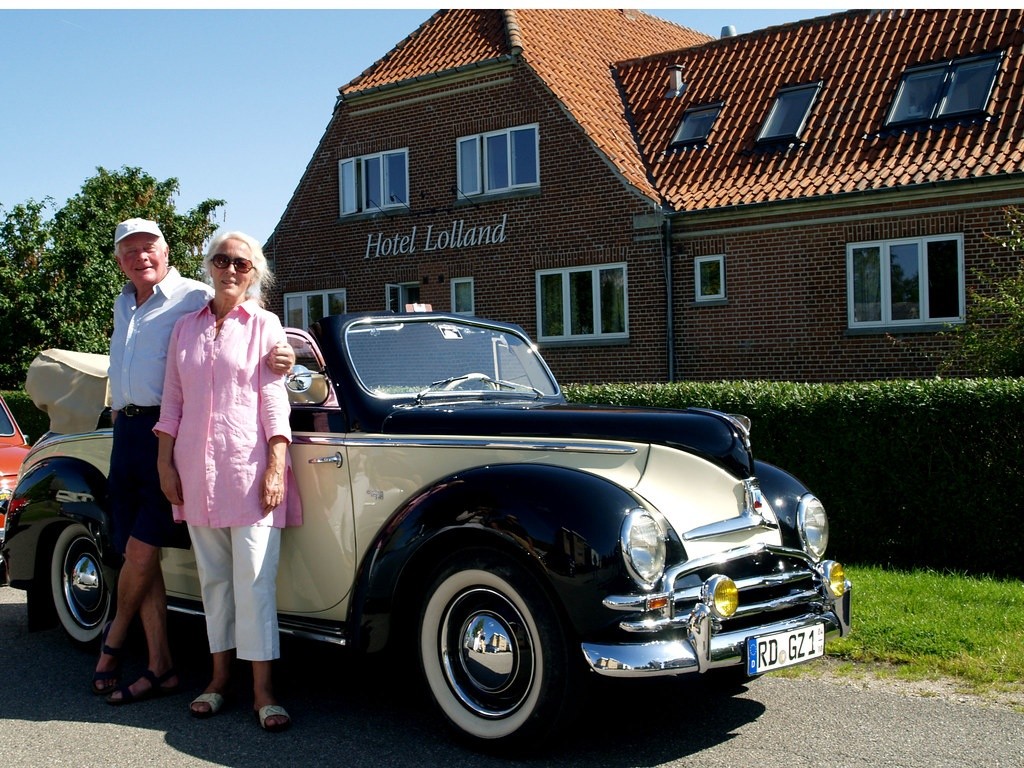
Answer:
[93,217,296,703]
[153,232,303,731]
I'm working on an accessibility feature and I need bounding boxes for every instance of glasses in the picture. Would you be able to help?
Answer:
[210,253,257,273]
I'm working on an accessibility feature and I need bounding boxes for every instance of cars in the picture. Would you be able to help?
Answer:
[4,301,854,758]
[0,391,35,571]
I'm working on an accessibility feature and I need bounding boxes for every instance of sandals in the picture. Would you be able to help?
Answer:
[89,620,125,696]
[104,667,180,705]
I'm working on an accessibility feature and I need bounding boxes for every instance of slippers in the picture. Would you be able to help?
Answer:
[252,699,292,733]
[188,690,231,720]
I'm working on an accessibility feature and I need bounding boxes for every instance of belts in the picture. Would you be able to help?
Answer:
[117,404,162,418]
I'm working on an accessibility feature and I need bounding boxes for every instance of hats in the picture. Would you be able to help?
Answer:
[114,217,162,247]
